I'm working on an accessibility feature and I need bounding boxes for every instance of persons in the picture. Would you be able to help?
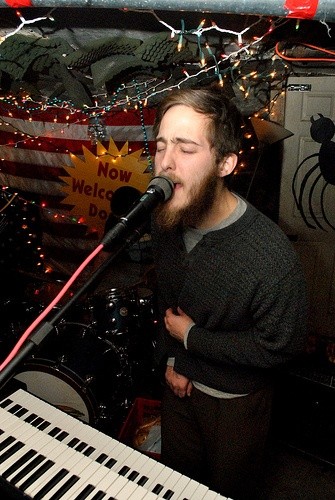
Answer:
[145,85,308,500]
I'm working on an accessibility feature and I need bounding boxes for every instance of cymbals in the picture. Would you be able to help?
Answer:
[74,273,142,290]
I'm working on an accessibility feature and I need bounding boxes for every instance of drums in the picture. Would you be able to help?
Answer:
[5,320,133,440]
[37,279,87,310]
[136,287,155,321]
[90,286,142,337]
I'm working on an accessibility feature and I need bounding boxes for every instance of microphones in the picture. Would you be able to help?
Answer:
[101,175,174,249]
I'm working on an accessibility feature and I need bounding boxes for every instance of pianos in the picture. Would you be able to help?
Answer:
[0,377,233,500]
